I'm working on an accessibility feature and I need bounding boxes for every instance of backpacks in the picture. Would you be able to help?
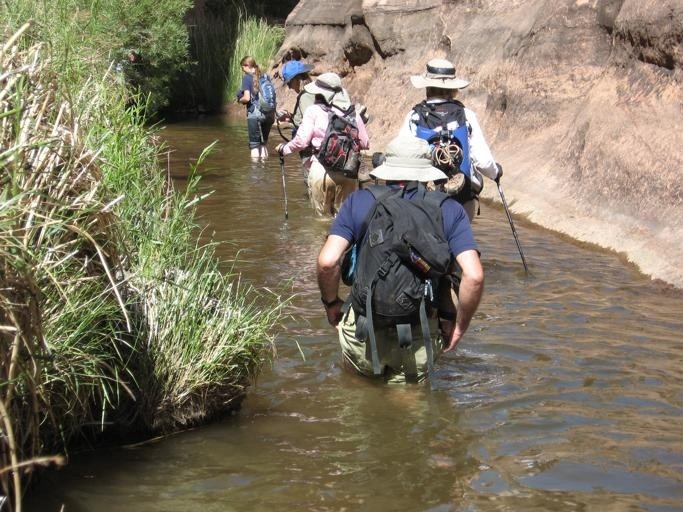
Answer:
[251,73,276,114]
[413,100,483,203]
[351,185,453,328]
[314,103,361,179]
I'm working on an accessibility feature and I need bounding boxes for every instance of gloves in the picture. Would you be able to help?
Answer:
[275,144,285,156]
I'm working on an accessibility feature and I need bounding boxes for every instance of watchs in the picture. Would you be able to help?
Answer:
[321,297,338,307]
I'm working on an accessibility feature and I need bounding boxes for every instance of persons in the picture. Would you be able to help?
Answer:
[275,73,371,221]
[373,59,503,224]
[275,60,315,183]
[237,57,276,163]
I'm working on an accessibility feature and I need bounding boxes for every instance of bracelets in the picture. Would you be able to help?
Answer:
[317,137,484,385]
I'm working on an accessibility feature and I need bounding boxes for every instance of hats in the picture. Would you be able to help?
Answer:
[411,59,471,89]
[369,135,449,181]
[282,62,315,86]
[304,73,351,111]
